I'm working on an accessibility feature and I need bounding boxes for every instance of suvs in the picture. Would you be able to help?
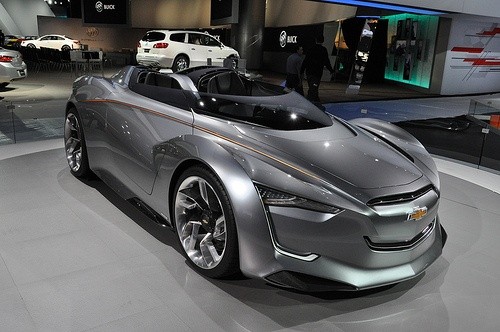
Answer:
[136,29,240,72]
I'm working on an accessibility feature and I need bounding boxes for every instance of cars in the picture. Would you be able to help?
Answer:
[20,34,82,51]
[0,46,27,86]
[3,34,33,49]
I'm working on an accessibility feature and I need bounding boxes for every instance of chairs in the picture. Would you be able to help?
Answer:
[138,71,254,116]
[20,46,114,73]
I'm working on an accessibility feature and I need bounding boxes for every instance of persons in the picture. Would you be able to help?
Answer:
[284,46,304,97]
[0,29,5,48]
[299,33,334,105]
[99,48,104,61]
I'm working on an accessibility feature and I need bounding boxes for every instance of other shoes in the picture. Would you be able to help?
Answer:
[318,106,327,111]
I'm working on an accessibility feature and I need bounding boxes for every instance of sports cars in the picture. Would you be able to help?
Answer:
[64,63,449,294]
